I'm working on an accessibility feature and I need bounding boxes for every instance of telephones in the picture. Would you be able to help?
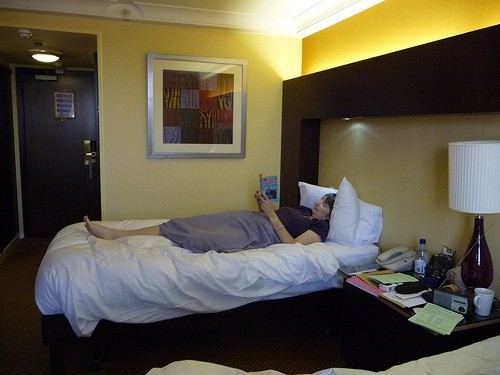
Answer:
[376,246,416,272]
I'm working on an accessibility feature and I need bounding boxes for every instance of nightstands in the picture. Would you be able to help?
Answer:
[336,262,500,372]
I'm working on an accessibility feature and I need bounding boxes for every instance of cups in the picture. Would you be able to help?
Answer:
[473,288,495,316]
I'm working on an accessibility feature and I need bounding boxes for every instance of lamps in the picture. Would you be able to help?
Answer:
[448,140,500,294]
[27,50,63,63]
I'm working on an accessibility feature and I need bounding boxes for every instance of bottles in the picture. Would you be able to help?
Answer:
[414,238,430,276]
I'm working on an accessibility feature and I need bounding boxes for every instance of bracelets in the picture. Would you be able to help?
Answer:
[274,225,285,230]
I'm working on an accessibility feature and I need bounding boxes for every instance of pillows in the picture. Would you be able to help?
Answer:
[296,176,383,248]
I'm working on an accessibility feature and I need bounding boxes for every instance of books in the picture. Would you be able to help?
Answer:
[260,176,278,202]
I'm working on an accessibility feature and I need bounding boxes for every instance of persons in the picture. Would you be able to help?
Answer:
[84,191,338,253]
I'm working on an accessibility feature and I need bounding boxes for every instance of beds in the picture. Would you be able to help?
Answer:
[34,176,384,375]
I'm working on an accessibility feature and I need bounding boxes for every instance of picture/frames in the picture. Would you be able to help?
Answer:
[146,53,247,159]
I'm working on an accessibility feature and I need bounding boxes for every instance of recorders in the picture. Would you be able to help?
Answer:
[433,222,495,315]
